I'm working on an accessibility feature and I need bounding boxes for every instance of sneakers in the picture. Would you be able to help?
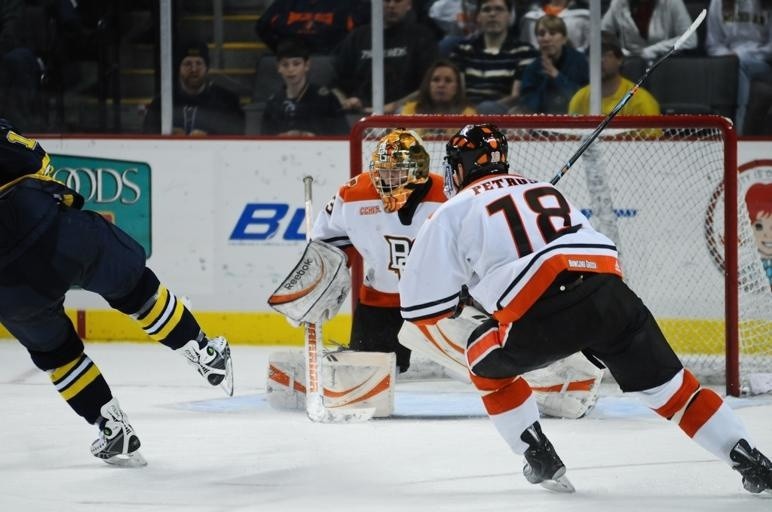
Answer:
[521,421,566,484]
[90,401,141,460]
[199,336,230,386]
[730,438,772,494]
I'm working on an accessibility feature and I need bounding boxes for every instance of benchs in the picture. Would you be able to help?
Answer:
[48,1,266,111]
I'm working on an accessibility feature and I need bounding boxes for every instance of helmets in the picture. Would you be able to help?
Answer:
[366,126,433,214]
[440,122,509,200]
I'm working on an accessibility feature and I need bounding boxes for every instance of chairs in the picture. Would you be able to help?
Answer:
[617,57,647,86]
[648,55,740,125]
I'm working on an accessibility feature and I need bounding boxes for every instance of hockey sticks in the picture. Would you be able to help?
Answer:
[302,175,376,423]
[552,9,707,185]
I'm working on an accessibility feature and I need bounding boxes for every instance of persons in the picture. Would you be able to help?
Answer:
[1,0,205,137]
[268,129,603,420]
[400,123,772,492]
[254,1,772,137]
[145,48,247,138]
[3,129,231,460]
